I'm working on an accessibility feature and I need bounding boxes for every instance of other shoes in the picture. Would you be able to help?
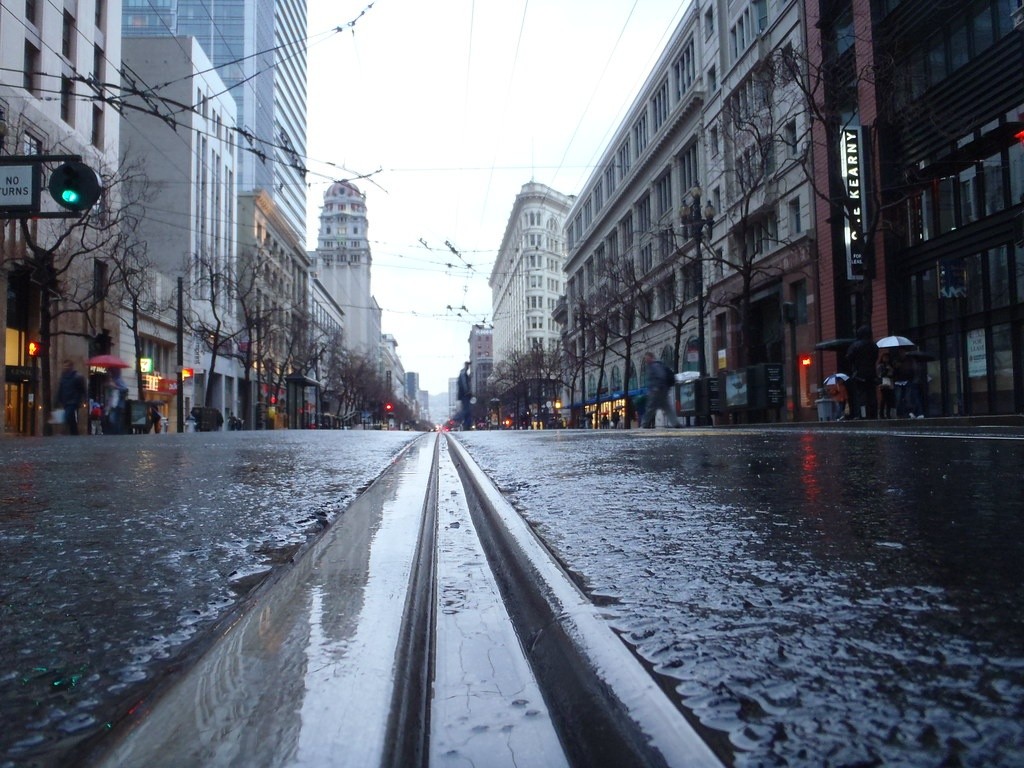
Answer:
[840,415,845,420]
[642,425,656,429]
[916,415,924,419]
[837,419,840,421]
[447,421,460,428]
[909,413,916,418]
[674,424,684,428]
[463,427,474,431]
[886,414,891,419]
[879,413,885,419]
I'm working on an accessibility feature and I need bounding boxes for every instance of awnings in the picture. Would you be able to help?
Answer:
[815,338,855,351]
[563,387,647,409]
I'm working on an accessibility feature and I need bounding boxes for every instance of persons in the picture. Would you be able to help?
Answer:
[848,354,925,419]
[103,368,128,433]
[445,362,474,431]
[639,352,681,428]
[53,360,84,435]
[602,414,608,429]
[90,402,106,435]
[612,410,619,428]
[831,378,849,421]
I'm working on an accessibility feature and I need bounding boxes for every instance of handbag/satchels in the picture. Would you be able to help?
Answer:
[879,376,894,391]
[610,421,614,427]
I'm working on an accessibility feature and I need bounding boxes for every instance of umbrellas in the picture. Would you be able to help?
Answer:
[875,335,914,353]
[823,373,849,385]
[88,355,131,369]
[598,412,608,415]
[905,350,935,362]
[614,406,622,410]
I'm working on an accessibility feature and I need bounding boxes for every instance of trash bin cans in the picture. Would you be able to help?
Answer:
[816,398,833,422]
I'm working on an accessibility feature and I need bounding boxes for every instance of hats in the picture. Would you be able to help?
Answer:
[465,362,470,364]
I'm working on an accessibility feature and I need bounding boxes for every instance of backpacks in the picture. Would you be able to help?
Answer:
[655,360,675,387]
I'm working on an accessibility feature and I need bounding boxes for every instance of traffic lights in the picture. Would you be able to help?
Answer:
[49,163,98,210]
[386,404,392,410]
[27,343,38,355]
